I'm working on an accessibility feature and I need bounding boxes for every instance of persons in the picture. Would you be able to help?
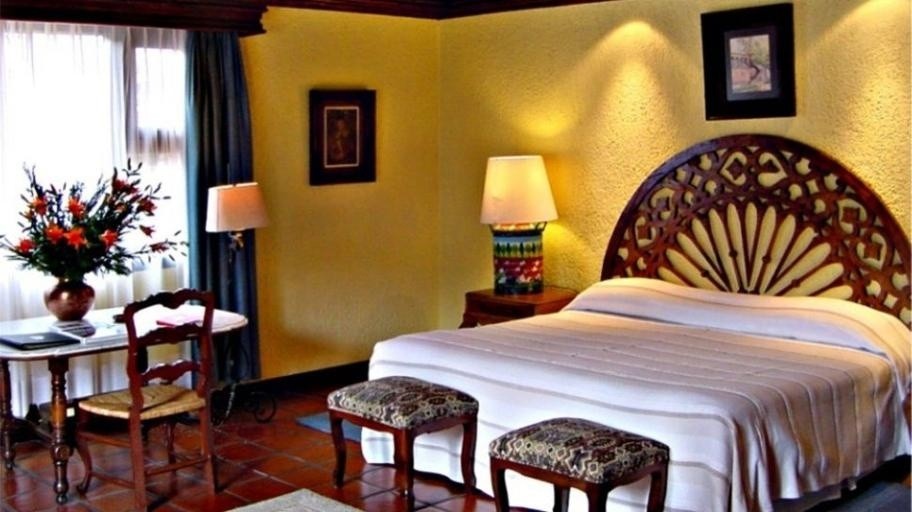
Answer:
[327,113,355,161]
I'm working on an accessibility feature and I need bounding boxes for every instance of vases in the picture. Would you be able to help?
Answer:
[44,277,96,319]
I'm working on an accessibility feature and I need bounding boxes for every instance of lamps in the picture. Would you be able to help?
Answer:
[205,180,277,423]
[479,154,560,294]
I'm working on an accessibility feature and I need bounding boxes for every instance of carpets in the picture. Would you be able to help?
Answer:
[296,411,362,443]
[218,486,367,512]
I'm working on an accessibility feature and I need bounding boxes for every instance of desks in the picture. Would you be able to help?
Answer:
[0,303,249,504]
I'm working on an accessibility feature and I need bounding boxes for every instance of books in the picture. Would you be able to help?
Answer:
[48,320,130,346]
[0,331,82,351]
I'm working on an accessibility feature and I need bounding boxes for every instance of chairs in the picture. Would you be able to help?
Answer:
[73,288,217,509]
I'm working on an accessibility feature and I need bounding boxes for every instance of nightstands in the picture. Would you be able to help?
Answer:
[458,286,578,329]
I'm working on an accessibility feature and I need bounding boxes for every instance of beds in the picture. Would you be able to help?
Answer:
[365,131,911,510]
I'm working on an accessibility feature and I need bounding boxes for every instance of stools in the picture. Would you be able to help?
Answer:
[486,417,671,509]
[329,375,480,507]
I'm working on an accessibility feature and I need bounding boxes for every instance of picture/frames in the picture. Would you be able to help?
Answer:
[698,3,799,121]
[308,87,377,187]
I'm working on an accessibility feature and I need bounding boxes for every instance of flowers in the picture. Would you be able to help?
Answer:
[0,160,191,278]
